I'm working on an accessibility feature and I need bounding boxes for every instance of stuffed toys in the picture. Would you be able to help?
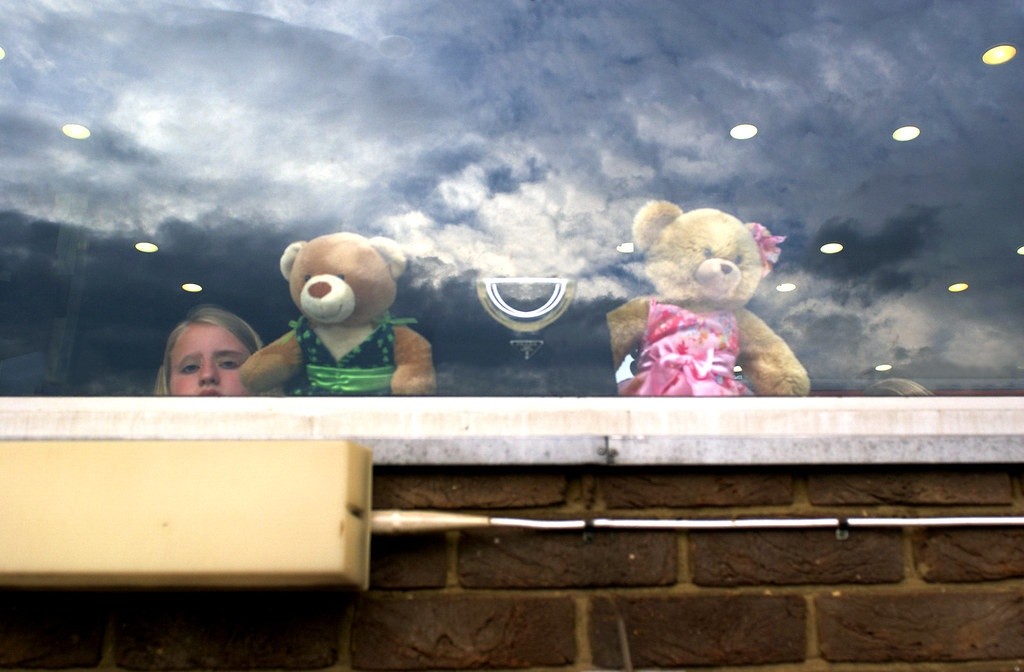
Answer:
[239,231,435,397]
[605,199,811,397]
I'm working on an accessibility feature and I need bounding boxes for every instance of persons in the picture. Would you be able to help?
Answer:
[154,304,285,396]
